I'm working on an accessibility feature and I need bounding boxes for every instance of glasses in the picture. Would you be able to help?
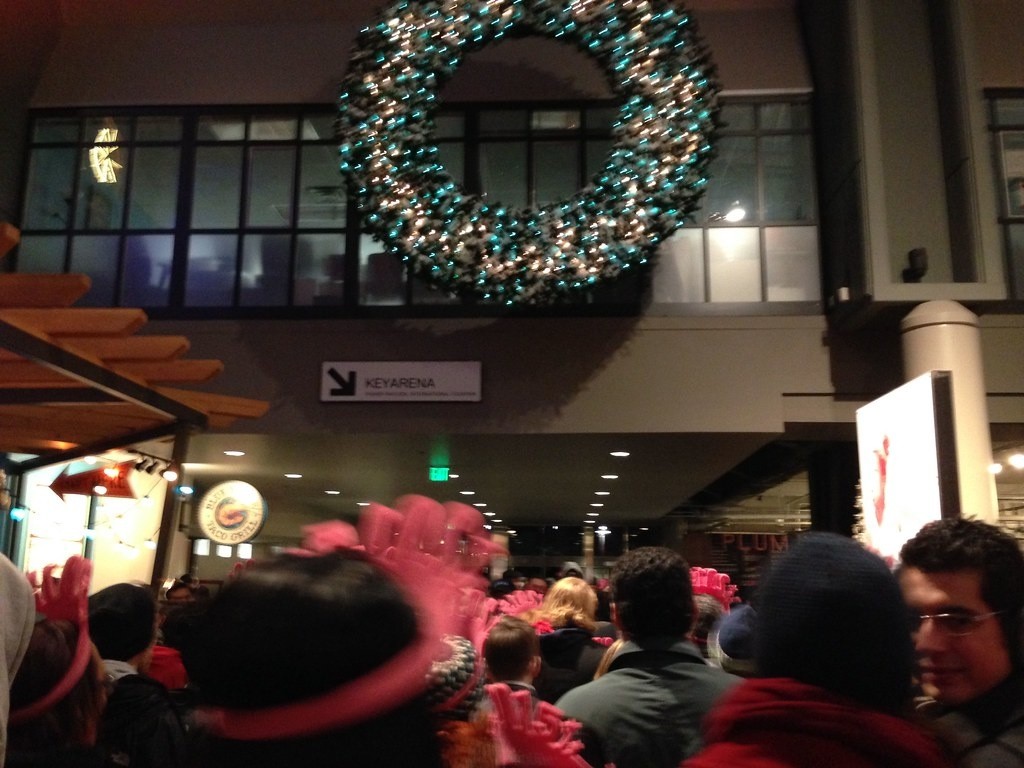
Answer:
[911,611,995,636]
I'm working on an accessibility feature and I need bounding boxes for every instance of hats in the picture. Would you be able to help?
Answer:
[715,606,759,674]
[88,583,155,661]
[739,531,916,687]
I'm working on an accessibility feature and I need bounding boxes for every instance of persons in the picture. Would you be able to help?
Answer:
[677,530,952,768]
[421,544,759,768]
[0,554,109,768]
[89,583,191,768]
[180,492,498,768]
[892,510,1024,768]
[122,571,215,728]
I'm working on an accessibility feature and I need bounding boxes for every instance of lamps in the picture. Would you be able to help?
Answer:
[159,462,172,478]
[135,455,151,472]
[145,457,160,475]
